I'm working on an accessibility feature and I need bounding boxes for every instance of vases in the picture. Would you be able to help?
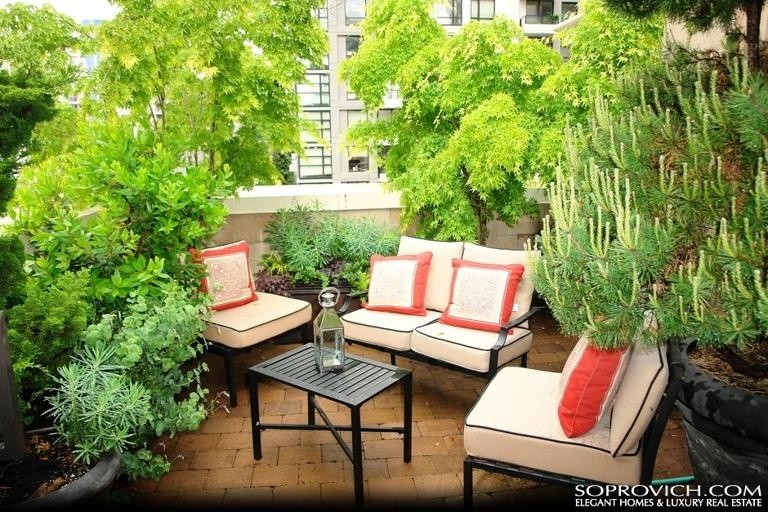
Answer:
[1,421,135,510]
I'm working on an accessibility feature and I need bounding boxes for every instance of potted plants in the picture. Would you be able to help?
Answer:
[517,44,768,512]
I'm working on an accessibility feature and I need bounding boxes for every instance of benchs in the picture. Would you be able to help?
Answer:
[335,281,549,394]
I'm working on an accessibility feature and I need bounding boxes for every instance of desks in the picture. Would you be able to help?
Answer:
[244,340,418,507]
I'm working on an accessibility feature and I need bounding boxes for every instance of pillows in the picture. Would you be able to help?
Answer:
[438,256,525,337]
[551,315,641,440]
[357,249,435,319]
[184,239,260,314]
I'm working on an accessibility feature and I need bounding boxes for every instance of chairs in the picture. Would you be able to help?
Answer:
[455,310,687,507]
[175,249,313,409]
